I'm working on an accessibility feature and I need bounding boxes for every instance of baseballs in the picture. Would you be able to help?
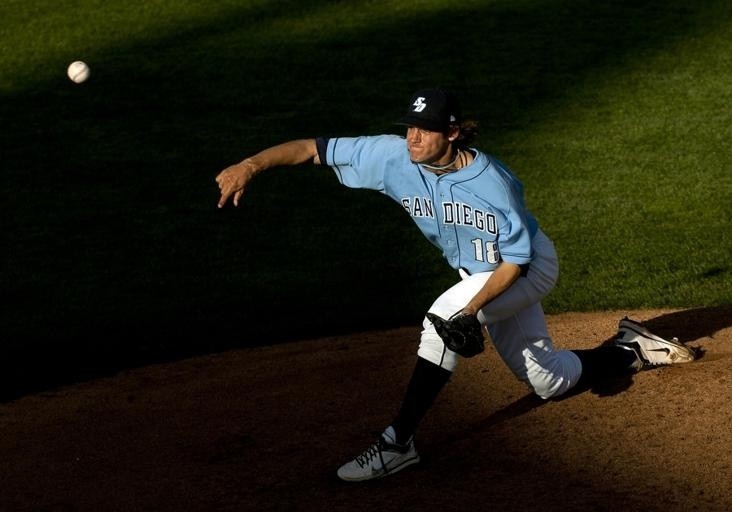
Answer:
[68,61,90,83]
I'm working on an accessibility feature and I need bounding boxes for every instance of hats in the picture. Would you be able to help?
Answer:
[392,90,463,134]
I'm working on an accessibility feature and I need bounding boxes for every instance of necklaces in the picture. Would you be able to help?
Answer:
[421,148,460,170]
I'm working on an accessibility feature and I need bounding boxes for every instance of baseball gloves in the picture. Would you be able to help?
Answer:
[425,312,484,357]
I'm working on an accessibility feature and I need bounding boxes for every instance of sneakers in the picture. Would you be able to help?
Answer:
[614,317,697,370]
[338,427,419,482]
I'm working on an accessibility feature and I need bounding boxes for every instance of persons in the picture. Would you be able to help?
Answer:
[214,89,697,483]
